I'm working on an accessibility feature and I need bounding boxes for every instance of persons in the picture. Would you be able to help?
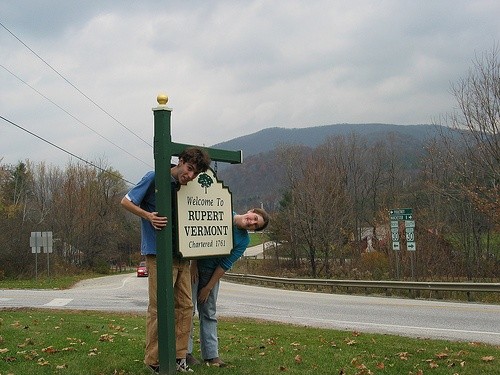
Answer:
[185,209,270,366]
[121,146,210,375]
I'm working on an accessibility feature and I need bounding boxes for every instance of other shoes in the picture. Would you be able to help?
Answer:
[146,364,159,375]
[176,359,194,373]
[204,358,226,368]
[186,353,202,367]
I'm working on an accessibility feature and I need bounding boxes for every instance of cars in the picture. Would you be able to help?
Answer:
[136,261,148,277]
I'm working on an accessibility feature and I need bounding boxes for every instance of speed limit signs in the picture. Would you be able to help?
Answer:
[392,232,399,243]
[406,233,414,241]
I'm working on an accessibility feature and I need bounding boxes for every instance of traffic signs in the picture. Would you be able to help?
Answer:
[390,208,412,221]
